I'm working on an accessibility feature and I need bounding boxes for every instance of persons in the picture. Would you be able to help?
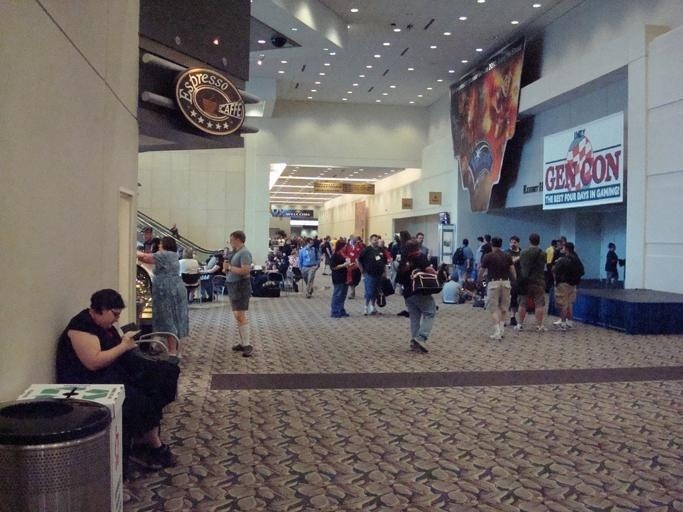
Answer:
[52,287,180,471]
[170,226,179,239]
[134,236,189,362]
[220,230,255,358]
[264,229,429,318]
[429,256,449,283]
[394,238,438,353]
[178,247,231,303]
[442,233,585,341]
[605,242,622,288]
[135,225,160,254]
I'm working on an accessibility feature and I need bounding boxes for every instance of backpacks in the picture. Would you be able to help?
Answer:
[119,344,181,406]
[452,247,465,265]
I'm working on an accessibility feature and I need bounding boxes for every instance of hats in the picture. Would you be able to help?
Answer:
[450,270,459,282]
[140,226,152,233]
[562,242,575,248]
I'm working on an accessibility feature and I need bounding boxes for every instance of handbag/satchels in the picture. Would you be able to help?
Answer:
[411,268,442,294]
[376,276,394,297]
[346,262,361,286]
[315,247,321,268]
[376,287,386,307]
[391,257,400,273]
[520,255,539,285]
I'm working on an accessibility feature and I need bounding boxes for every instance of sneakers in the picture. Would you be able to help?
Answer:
[553,320,574,331]
[513,324,525,335]
[535,326,548,335]
[305,287,313,298]
[370,310,382,316]
[202,298,208,302]
[410,337,429,354]
[208,296,215,302]
[363,310,369,316]
[331,311,349,318]
[129,446,175,469]
[232,344,244,351]
[242,346,254,356]
[490,331,505,340]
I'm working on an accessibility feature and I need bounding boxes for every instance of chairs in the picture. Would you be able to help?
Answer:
[120,323,179,361]
[181,272,203,305]
[211,275,227,303]
[267,272,284,290]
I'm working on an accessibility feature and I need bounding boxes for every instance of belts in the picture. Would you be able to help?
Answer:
[489,276,511,282]
[302,264,316,268]
[225,277,250,285]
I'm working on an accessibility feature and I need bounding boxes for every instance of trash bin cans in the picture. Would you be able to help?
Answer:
[0,399,111,512]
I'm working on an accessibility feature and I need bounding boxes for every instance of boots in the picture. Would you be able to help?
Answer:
[510,317,519,326]
